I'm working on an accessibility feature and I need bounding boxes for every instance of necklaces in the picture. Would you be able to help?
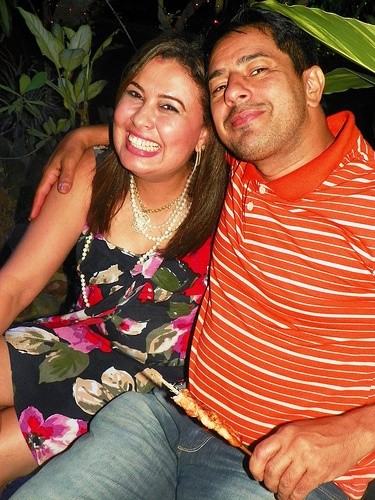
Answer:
[75,152,202,315]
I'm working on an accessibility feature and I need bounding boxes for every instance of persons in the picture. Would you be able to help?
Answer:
[0,37,234,485]
[3,5,374,500]
